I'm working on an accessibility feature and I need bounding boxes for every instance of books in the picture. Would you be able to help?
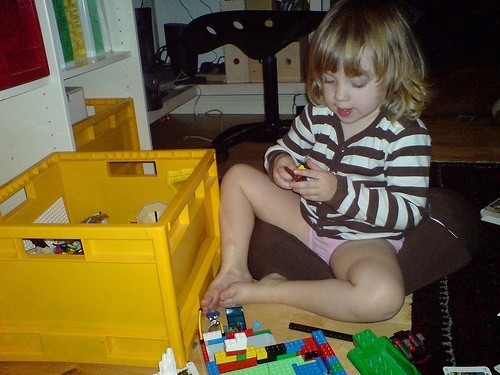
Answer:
[480,197,500,225]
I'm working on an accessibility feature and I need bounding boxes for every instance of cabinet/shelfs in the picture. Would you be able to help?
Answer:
[0,0,166,252]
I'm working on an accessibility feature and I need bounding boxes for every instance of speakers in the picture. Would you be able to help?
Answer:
[163,23,198,75]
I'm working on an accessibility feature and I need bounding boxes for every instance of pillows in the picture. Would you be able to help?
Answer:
[218,142,471,298]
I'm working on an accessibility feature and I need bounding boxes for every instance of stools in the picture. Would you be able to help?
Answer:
[183,10,327,162]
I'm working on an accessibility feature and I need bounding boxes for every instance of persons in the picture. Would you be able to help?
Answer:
[199,0,431,323]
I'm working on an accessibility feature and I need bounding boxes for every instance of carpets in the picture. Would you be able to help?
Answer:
[188,160,500,375]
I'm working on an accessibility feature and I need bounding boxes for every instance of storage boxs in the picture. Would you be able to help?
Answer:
[66,87,88,124]
[72,98,145,176]
[0,148,223,368]
[220,1,309,85]
